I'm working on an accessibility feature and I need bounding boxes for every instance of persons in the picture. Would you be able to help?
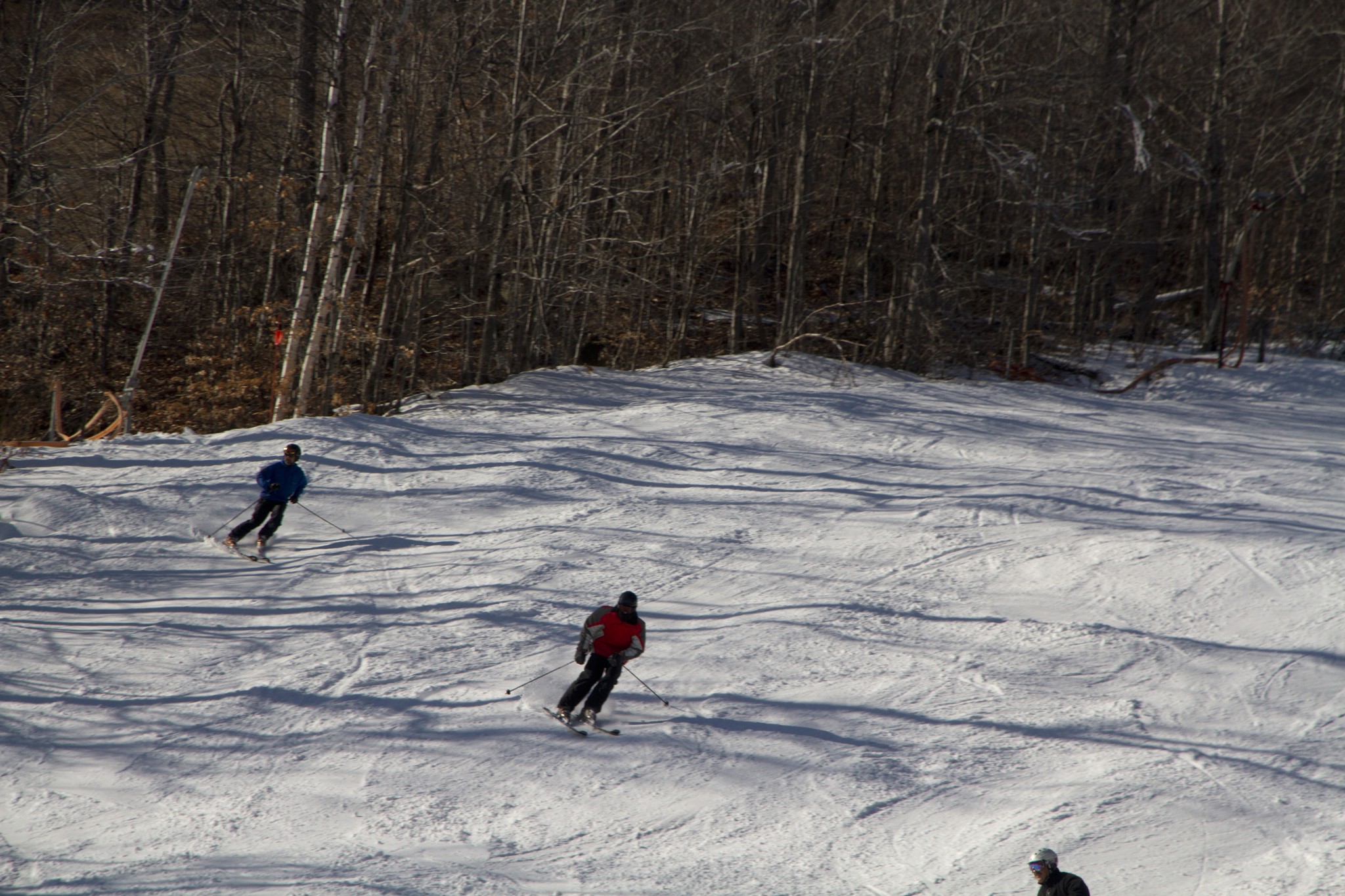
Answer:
[225,444,307,556]
[1027,848,1090,896]
[554,591,646,724]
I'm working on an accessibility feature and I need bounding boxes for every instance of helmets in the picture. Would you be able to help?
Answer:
[619,591,637,607]
[285,444,300,459]
[1028,848,1058,868]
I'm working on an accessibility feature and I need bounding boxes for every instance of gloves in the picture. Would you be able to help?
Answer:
[290,495,298,503]
[270,483,281,491]
[608,654,622,666]
[575,651,585,665]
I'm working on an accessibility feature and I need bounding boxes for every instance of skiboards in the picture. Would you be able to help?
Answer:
[203,533,270,563]
[542,706,621,737]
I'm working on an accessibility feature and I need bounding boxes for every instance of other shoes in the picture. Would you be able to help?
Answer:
[257,538,265,553]
[581,707,596,724]
[226,536,236,547]
[558,706,570,724]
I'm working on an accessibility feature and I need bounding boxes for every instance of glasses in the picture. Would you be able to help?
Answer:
[284,447,296,455]
[1029,861,1045,873]
